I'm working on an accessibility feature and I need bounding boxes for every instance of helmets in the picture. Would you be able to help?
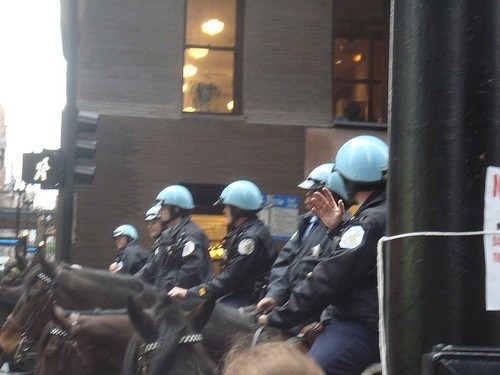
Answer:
[144,205,161,221]
[296,162,348,203]
[330,135,389,183]
[212,180,264,211]
[112,224,138,240]
[154,185,195,209]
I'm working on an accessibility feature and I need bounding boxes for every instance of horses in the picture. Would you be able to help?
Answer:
[1,243,325,375]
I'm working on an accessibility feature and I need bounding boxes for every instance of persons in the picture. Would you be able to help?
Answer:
[109,225,152,276]
[256,135,389,375]
[225,340,325,375]
[133,188,214,291]
[167,180,276,308]
